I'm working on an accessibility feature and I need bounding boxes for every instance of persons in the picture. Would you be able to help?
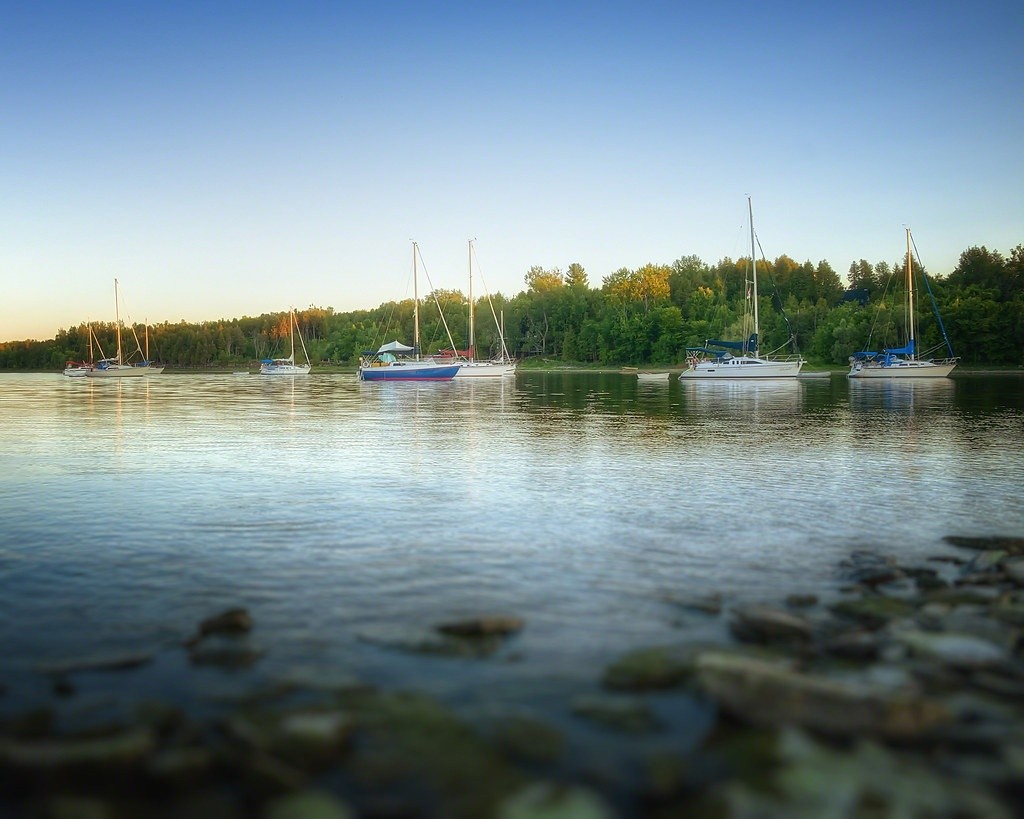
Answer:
[849,354,855,373]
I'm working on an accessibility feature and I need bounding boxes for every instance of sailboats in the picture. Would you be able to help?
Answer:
[84,277,150,376]
[847,227,960,376]
[63,320,111,377]
[424,239,517,377]
[680,196,808,378]
[359,240,462,381]
[129,317,165,373]
[493,308,516,376]
[260,304,311,374]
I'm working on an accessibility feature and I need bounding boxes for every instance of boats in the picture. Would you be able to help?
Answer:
[636,371,669,380]
[800,371,832,378]
